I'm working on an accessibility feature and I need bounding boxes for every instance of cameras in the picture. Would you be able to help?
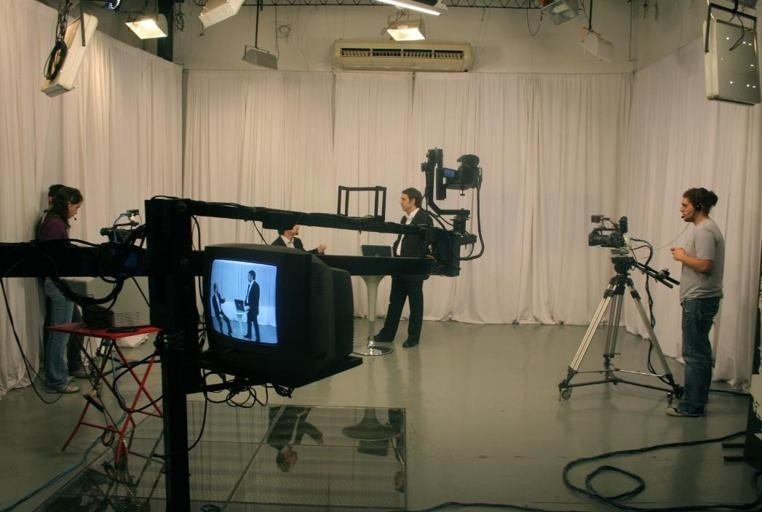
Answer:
[589,215,627,247]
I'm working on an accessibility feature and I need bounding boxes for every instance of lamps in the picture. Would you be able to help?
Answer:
[579,1,621,61]
[381,14,426,42]
[241,1,281,74]
[377,1,444,16]
[199,0,247,31]
[124,14,169,40]
[541,1,576,26]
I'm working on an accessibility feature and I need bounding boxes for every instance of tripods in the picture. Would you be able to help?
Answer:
[558,256,683,405]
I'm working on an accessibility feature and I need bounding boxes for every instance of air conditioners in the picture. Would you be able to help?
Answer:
[331,34,476,72]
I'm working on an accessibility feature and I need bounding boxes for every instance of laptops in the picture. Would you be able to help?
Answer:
[362,245,391,256]
[234,299,248,311]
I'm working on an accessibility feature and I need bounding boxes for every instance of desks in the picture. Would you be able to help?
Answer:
[353,269,395,356]
[48,320,167,469]
[341,408,390,440]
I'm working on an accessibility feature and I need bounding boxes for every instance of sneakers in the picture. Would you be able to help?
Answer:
[68,366,90,378]
[44,380,80,394]
[666,403,703,417]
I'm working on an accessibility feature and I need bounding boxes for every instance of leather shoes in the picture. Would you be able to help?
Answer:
[243,334,252,339]
[367,333,395,342]
[255,339,260,342]
[402,338,419,348]
[228,332,233,337]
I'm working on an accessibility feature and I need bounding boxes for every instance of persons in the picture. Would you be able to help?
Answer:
[243,268,263,344]
[267,217,331,255]
[38,185,86,397]
[264,404,324,473]
[36,185,94,381]
[211,281,234,341]
[661,187,730,418]
[386,408,407,493]
[362,184,435,347]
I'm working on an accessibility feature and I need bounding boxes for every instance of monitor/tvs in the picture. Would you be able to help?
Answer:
[203,242,354,377]
[59,276,151,330]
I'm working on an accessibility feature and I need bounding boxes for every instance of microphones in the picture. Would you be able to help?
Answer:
[681,208,696,218]
[73,215,77,220]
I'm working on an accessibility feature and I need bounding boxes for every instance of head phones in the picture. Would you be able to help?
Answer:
[695,188,702,211]
